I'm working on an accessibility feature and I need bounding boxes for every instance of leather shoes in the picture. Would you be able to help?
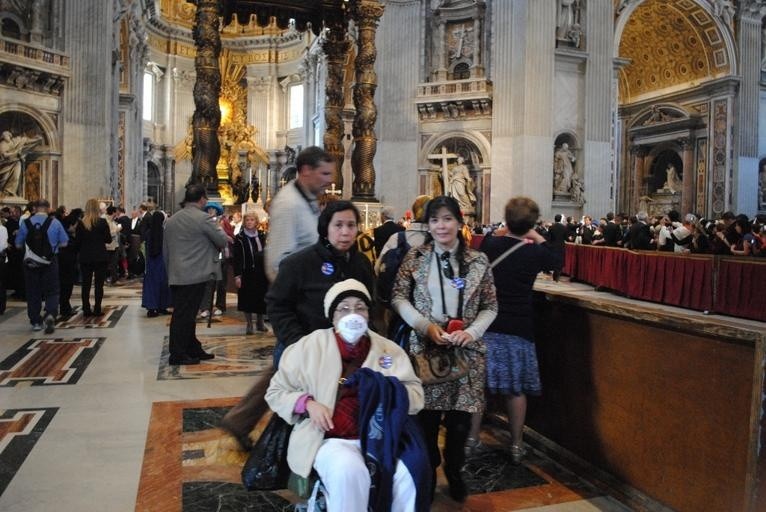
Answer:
[198,350,215,360]
[169,356,199,365]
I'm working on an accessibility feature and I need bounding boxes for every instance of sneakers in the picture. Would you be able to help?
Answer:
[201,311,209,318]
[214,310,224,316]
[44,316,55,335]
[33,322,44,331]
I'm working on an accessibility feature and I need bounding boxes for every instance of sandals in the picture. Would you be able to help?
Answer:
[510,443,526,465]
[464,437,488,457]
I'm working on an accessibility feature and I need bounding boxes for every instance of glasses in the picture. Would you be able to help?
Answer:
[441,251,456,280]
[334,305,368,315]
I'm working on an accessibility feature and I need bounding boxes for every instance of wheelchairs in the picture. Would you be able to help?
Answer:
[284,407,441,511]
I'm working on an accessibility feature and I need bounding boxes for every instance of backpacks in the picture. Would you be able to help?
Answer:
[23,216,54,270]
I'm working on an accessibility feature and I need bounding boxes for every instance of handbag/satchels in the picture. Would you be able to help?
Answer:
[414,343,472,386]
[240,411,294,491]
[376,231,412,309]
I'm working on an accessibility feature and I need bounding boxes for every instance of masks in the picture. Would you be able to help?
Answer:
[336,313,367,344]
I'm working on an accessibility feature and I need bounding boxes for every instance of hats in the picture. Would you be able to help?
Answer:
[323,278,372,320]
[204,202,224,216]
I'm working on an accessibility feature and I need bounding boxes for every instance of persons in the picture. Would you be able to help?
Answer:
[462,198,554,464]
[273,201,379,373]
[535,209,579,282]
[0,131,41,194]
[390,196,497,503]
[465,221,505,254]
[554,143,575,192]
[162,184,226,363]
[265,279,429,512]
[576,209,765,258]
[370,196,431,331]
[448,158,475,224]
[664,165,680,194]
[0,197,273,335]
[374,206,414,258]
[218,144,335,453]
